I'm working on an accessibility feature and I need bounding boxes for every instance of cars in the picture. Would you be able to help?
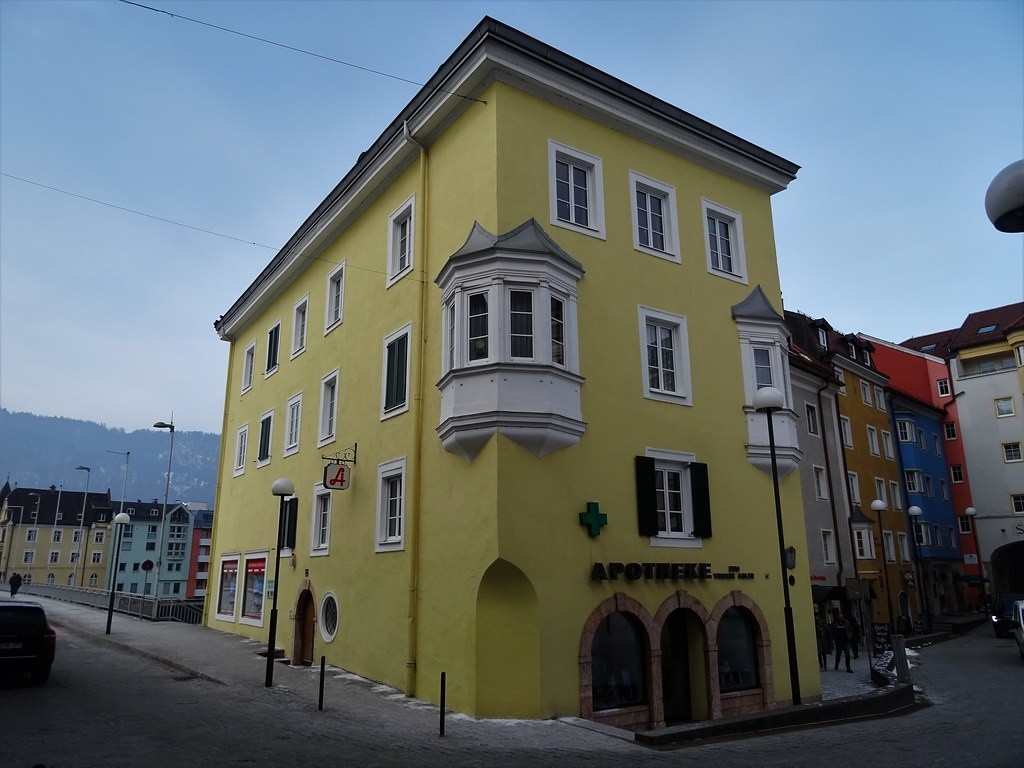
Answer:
[992,594,1024,639]
[1011,600,1024,659]
[0,599,56,691]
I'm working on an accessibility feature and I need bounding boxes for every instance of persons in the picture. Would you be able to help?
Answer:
[815,616,834,672]
[9,573,20,598]
[846,616,861,659]
[17,574,22,587]
[831,613,854,673]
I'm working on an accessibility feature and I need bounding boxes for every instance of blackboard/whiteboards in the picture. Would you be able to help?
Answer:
[873,623,890,654]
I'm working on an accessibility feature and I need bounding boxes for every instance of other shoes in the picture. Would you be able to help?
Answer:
[846,664,854,673]
[834,662,839,670]
[853,654,860,660]
[820,663,826,671]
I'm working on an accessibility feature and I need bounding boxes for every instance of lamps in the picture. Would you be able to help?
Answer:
[785,546,796,570]
[1001,529,1006,532]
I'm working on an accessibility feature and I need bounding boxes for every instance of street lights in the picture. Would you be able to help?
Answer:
[106,512,130,635]
[870,500,896,635]
[25,492,41,585]
[7,505,24,576]
[908,506,934,633]
[4,518,19,581]
[264,478,294,687]
[44,484,63,586]
[153,422,175,598]
[81,520,93,587]
[965,507,991,619]
[72,465,91,586]
[106,450,131,590]
[753,387,802,706]
